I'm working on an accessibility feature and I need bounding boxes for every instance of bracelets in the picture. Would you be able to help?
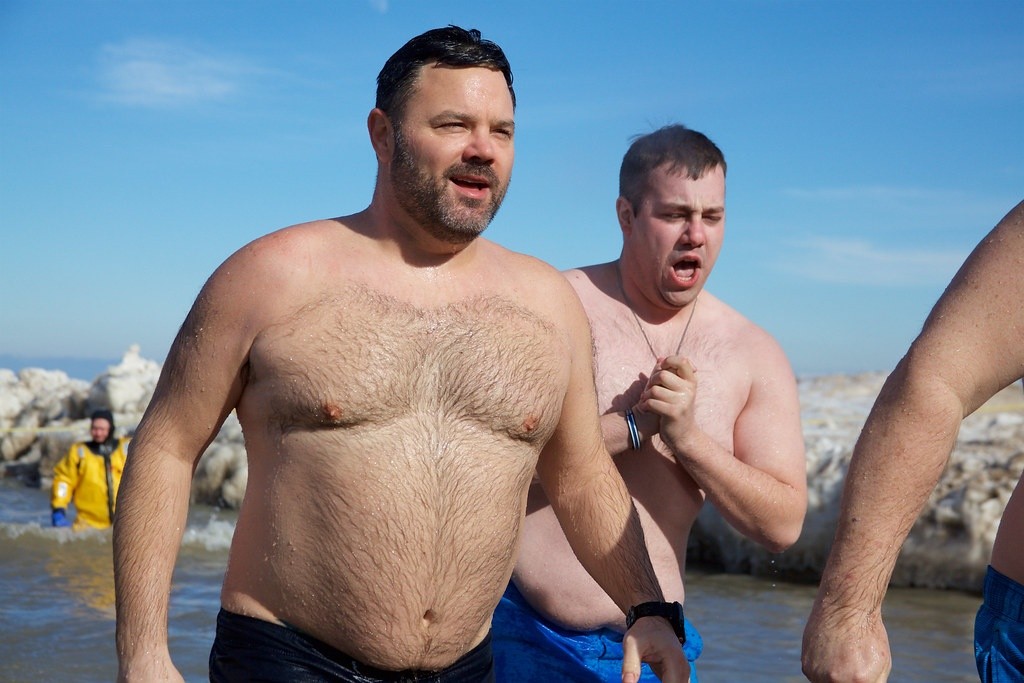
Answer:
[625,407,639,451]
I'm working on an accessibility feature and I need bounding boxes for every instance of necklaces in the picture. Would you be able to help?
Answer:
[614,262,697,370]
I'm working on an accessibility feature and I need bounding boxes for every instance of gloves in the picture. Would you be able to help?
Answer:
[51,508,72,529]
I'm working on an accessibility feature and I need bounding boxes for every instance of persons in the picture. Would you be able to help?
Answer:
[50,410,129,531]
[795,198,1024,683]
[110,25,695,682]
[487,120,808,681]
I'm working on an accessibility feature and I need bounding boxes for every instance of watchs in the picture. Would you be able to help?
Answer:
[624,599,687,646]
